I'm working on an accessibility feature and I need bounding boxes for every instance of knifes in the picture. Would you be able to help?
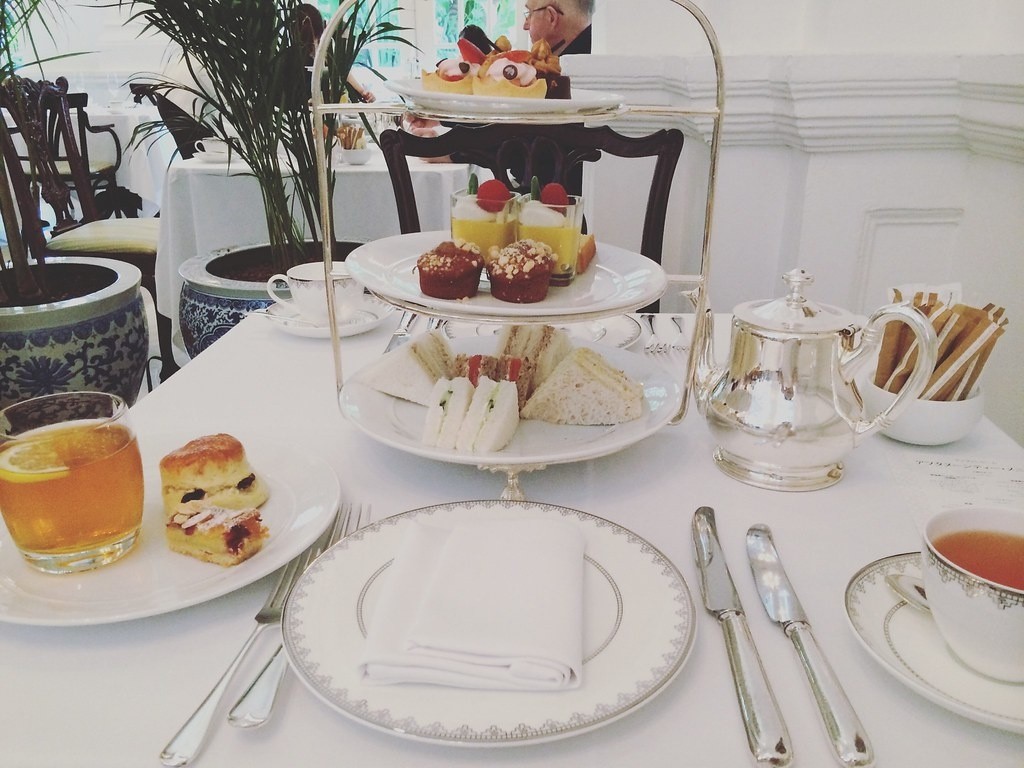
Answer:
[746,522,876,768]
[691,506,794,767]
[384,311,420,354]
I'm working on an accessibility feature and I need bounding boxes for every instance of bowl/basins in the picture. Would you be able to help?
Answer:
[342,149,371,165]
[863,370,987,448]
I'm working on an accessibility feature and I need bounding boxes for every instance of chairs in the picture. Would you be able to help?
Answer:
[376,125,689,313]
[1,74,186,382]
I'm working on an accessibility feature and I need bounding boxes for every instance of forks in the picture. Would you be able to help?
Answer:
[670,316,692,352]
[640,315,671,355]
[157,501,374,768]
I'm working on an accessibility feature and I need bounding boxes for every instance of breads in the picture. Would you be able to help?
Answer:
[165,502,266,567]
[157,432,270,513]
[575,233,597,274]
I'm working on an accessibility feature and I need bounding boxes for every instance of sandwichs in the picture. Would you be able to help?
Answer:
[350,321,646,452]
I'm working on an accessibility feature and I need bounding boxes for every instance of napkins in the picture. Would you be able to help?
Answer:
[361,525,583,690]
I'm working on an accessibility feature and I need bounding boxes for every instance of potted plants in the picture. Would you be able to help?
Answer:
[82,0,424,355]
[0,1,153,428]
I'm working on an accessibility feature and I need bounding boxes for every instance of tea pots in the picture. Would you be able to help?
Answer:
[678,268,940,493]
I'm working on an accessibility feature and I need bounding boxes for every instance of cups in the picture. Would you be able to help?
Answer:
[194,136,237,152]
[266,261,364,324]
[1,391,146,575]
[449,187,520,268]
[921,504,1024,684]
[514,192,585,287]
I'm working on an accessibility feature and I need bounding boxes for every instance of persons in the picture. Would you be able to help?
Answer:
[291,4,376,104]
[406,0,596,197]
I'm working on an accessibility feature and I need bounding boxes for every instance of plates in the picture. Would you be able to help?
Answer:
[264,295,396,339]
[441,314,642,349]
[339,337,681,464]
[842,551,1023,736]
[279,498,698,750]
[384,78,626,112]
[0,430,343,627]
[192,152,241,162]
[346,230,667,315]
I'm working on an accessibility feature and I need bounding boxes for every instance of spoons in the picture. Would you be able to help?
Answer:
[884,573,932,618]
[243,311,344,327]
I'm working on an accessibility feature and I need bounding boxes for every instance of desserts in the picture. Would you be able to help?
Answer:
[450,173,580,279]
[420,25,572,100]
[487,240,560,304]
[412,240,485,300]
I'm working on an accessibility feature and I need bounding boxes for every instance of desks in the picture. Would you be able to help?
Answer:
[1,290,1024,768]
[155,137,492,366]
[0,95,179,242]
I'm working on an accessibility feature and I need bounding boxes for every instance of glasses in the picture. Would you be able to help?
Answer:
[523,4,564,20]
